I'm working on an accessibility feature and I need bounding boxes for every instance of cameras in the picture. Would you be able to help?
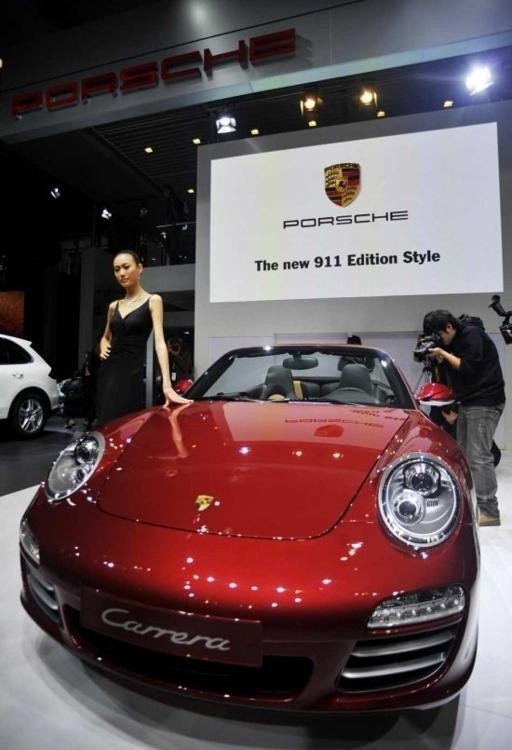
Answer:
[411,332,445,363]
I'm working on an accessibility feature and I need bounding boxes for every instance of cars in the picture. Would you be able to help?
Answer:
[18,345,481,714]
[0,334,61,437]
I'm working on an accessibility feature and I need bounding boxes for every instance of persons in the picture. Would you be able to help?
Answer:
[418,309,506,527]
[82,347,100,430]
[167,336,192,387]
[338,335,375,374]
[93,249,196,428]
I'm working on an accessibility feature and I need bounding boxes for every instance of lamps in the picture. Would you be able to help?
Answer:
[215,109,238,134]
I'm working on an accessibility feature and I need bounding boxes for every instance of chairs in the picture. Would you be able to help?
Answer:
[321,364,387,403]
[257,382,321,400]
[258,365,299,400]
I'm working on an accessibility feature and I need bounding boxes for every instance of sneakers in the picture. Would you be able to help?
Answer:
[478,512,500,525]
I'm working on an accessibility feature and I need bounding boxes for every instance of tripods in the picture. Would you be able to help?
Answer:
[412,363,432,393]
